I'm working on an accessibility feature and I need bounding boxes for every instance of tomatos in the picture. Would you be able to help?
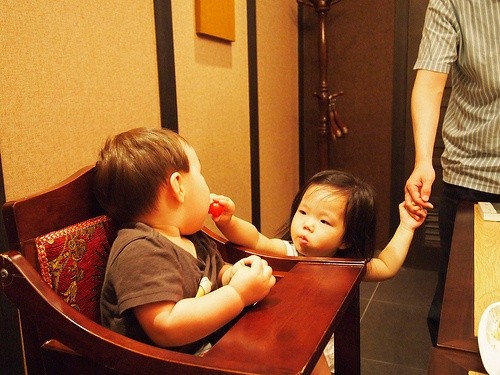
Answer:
[209,202,222,217]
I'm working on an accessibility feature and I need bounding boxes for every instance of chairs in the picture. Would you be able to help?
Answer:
[0,163,366,375]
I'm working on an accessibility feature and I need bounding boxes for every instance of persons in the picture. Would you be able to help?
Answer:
[403,0,499,347]
[209,170,425,375]
[92,126,333,375]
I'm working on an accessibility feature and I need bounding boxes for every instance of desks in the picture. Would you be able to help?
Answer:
[426,200,499,374]
